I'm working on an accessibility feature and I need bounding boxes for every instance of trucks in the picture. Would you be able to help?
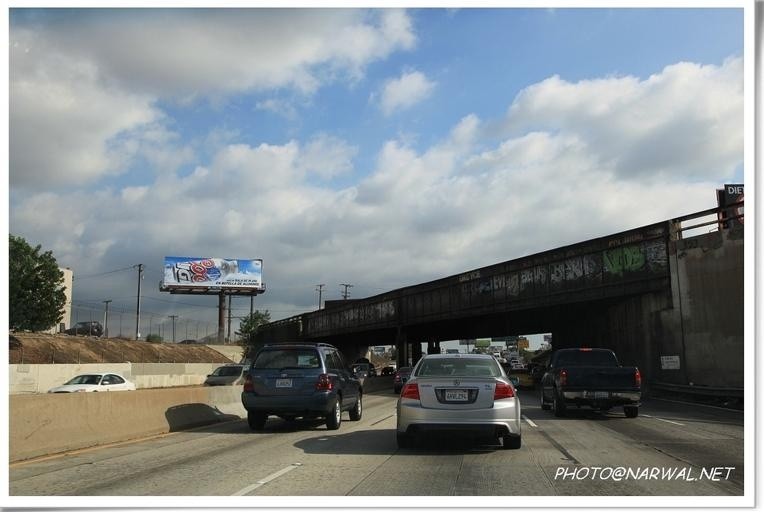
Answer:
[350,363,377,377]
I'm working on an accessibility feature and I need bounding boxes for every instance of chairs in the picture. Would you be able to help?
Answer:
[425,361,492,375]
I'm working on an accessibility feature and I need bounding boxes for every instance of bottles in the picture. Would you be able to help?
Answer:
[522,272,533,290]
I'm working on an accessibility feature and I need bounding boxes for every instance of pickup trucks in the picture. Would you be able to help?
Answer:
[539,349,641,420]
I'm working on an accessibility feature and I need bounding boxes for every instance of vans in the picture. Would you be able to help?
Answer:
[205,365,251,385]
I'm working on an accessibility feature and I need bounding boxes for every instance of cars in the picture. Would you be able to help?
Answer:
[242,343,363,430]
[48,374,137,392]
[397,354,521,453]
[381,366,396,376]
[506,369,535,390]
[393,367,414,394]
[494,352,528,368]
